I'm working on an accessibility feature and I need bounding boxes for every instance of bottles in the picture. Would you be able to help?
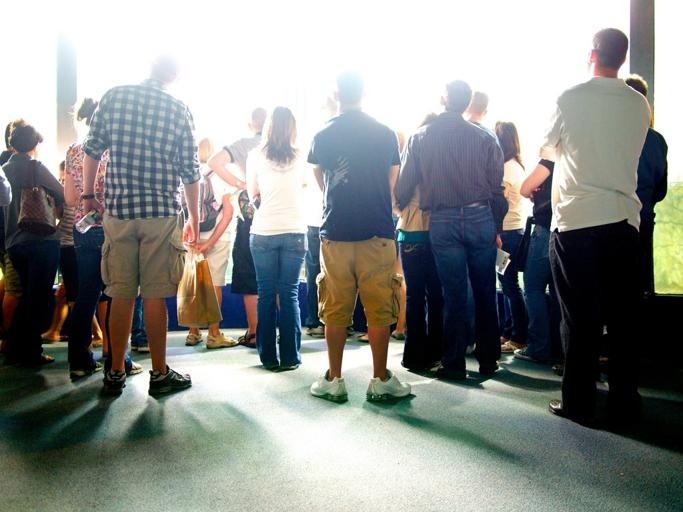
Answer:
[75,210,99,235]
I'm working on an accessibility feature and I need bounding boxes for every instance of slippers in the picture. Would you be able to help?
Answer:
[14,352,56,366]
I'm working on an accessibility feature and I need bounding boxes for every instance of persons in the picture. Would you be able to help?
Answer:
[1,27,199,395]
[184,103,324,373]
[308,72,413,405]
[393,82,527,385]
[520,27,668,421]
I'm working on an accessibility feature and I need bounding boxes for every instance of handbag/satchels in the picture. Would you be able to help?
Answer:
[174,256,223,331]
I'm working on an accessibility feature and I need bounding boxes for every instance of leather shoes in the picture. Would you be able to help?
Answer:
[605,387,641,430]
[355,333,371,342]
[391,329,405,341]
[547,397,579,421]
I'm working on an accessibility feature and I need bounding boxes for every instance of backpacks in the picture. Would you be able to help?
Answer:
[178,170,217,232]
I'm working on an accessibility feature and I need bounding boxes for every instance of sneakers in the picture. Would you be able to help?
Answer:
[91,336,102,349]
[16,186,58,236]
[67,362,102,379]
[310,369,348,404]
[303,325,324,339]
[102,372,126,394]
[347,327,355,334]
[130,342,136,350]
[137,344,149,353]
[147,365,192,397]
[366,368,412,401]
[479,359,500,376]
[436,363,468,381]
[512,347,537,363]
[40,333,60,345]
[126,361,143,376]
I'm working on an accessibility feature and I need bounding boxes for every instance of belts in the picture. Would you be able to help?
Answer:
[433,201,491,210]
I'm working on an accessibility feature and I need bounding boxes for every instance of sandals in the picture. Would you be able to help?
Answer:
[498,339,524,353]
[185,333,203,345]
[206,334,240,349]
[238,330,257,348]
